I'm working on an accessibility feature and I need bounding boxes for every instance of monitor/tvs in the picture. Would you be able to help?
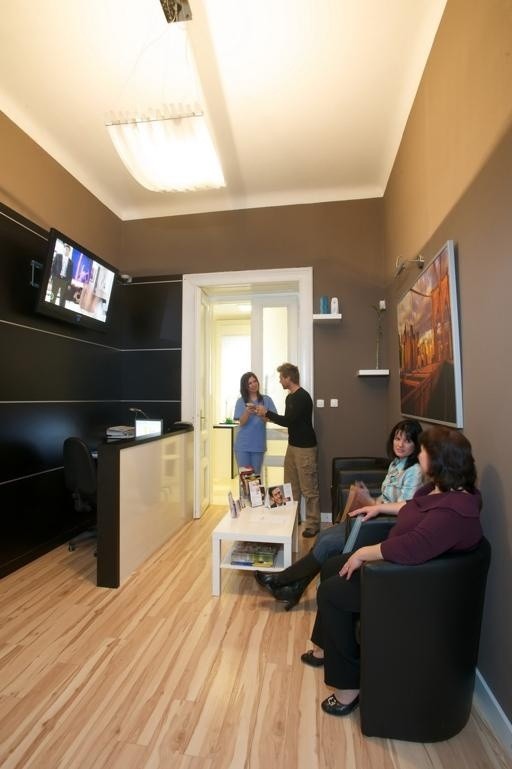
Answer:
[36,226,119,333]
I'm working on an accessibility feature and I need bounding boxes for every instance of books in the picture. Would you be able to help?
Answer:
[230,542,279,568]
[106,425,135,439]
[239,464,266,508]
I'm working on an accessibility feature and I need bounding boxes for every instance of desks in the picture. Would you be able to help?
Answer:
[213,422,239,480]
[211,501,298,597]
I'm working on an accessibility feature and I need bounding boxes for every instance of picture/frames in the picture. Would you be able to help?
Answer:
[396,240,464,429]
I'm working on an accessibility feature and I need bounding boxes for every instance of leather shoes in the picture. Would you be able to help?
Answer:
[301,650,325,667]
[321,693,360,715]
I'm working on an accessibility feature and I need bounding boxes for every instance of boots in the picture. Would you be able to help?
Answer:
[266,566,321,611]
[253,551,320,593]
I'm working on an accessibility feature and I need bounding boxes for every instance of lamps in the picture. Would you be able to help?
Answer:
[106,105,226,193]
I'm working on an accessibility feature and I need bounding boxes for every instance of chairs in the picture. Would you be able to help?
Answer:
[62,438,98,558]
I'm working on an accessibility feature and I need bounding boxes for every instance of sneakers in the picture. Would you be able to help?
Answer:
[302,528,318,537]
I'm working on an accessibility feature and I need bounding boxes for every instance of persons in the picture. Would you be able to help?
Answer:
[253,418,425,612]
[268,487,286,509]
[231,371,278,476]
[49,245,73,308]
[257,361,321,538]
[300,424,482,717]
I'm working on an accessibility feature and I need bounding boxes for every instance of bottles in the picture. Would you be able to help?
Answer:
[320,296,328,314]
[331,297,339,315]
